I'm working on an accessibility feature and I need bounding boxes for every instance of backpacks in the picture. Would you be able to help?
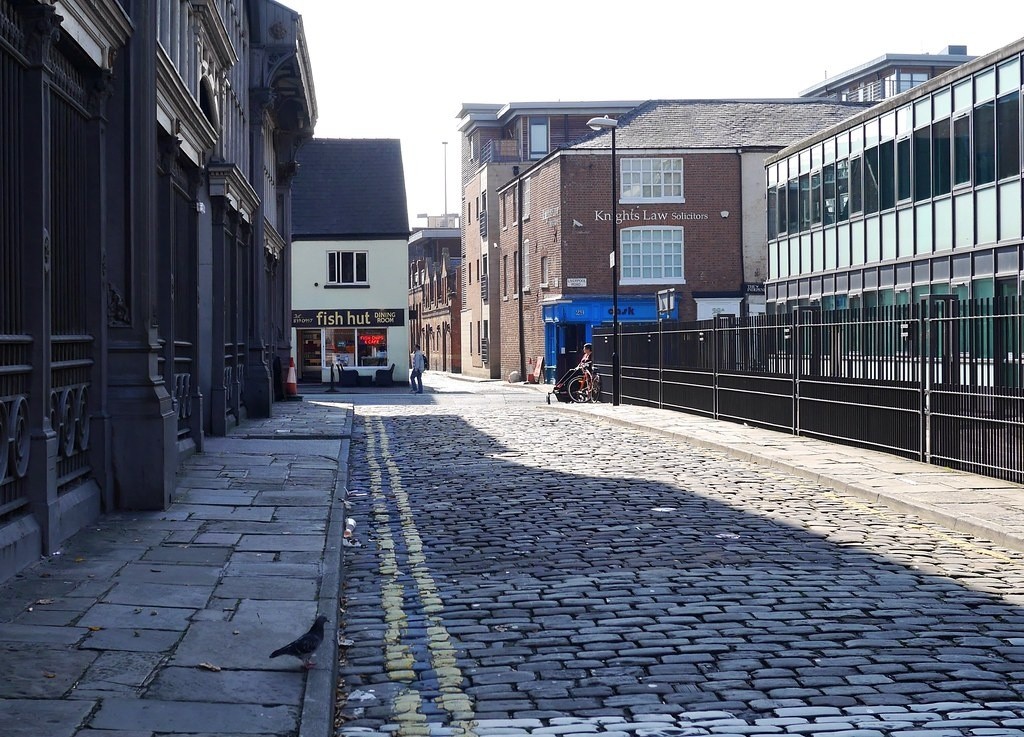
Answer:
[422,355,427,368]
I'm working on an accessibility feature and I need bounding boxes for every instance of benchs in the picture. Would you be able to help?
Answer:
[374,364,395,385]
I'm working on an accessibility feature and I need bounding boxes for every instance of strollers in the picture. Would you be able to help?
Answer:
[547,366,582,404]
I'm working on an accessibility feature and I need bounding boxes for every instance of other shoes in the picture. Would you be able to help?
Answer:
[416,391,422,394]
[411,389,417,391]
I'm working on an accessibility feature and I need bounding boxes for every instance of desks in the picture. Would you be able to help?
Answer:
[357,376,372,389]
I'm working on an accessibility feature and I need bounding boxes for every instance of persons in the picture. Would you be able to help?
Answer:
[409,344,424,394]
[574,343,595,403]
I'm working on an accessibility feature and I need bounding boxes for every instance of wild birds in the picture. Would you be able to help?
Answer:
[267,615,330,670]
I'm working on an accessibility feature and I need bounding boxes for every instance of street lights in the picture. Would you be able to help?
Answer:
[587,115,620,407]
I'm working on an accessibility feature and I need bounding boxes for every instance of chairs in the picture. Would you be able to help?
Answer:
[336,364,358,386]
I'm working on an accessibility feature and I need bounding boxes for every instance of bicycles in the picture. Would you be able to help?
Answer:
[567,360,601,404]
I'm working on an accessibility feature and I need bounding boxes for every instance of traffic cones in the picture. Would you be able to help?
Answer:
[286,356,298,395]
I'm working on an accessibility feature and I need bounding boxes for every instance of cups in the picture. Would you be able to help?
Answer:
[346,518,356,531]
